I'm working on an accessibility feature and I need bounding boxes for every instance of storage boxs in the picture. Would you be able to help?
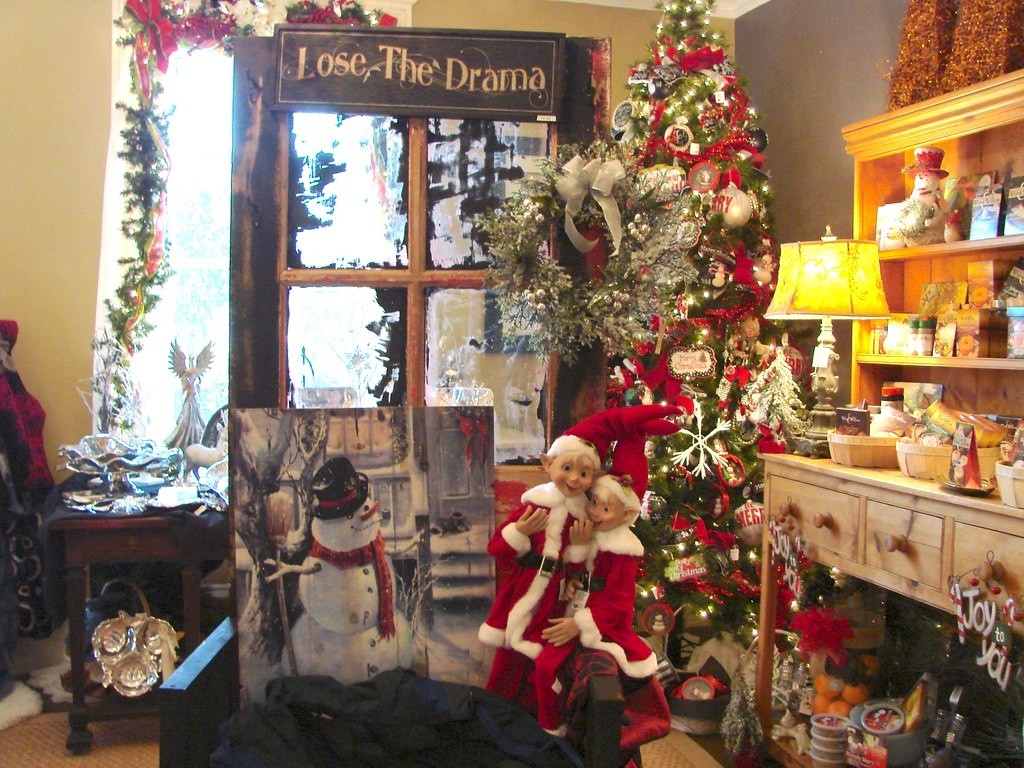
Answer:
[836,408,871,437]
[931,175,1024,359]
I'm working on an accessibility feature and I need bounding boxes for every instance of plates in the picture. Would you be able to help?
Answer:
[935,476,995,496]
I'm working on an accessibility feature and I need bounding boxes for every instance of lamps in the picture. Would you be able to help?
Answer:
[763,223,891,460]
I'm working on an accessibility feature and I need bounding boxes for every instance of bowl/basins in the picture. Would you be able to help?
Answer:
[848,698,929,767]
[994,461,1024,510]
[828,431,902,468]
[976,446,1001,481]
[809,715,849,767]
[895,439,954,479]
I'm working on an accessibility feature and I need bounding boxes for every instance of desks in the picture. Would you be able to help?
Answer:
[47,460,228,757]
[754,451,1024,768]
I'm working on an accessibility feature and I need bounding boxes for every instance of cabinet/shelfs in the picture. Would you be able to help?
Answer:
[776,68,1024,417]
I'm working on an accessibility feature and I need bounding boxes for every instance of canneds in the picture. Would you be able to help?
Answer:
[918,320,934,356]
[881,387,904,414]
[908,320,919,355]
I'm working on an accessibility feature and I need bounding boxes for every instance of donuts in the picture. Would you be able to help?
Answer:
[958,336,974,355]
[971,288,988,306]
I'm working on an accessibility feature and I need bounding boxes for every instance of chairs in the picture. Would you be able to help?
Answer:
[158,612,626,767]
